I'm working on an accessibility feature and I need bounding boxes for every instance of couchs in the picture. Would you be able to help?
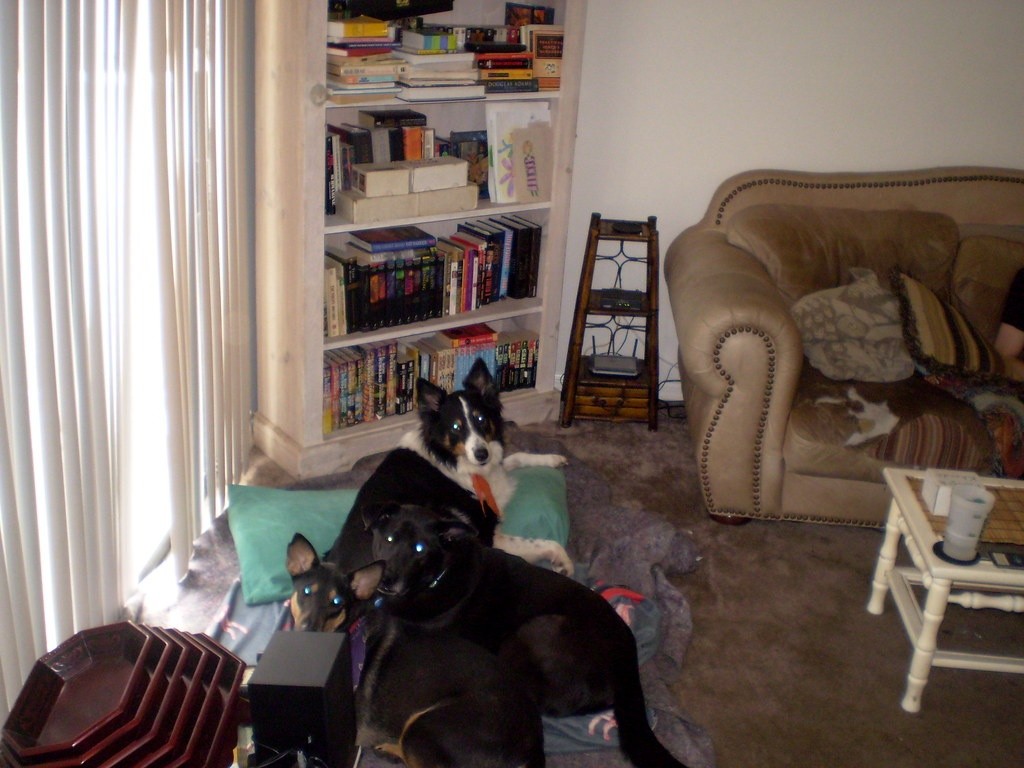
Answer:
[663,164,1024,526]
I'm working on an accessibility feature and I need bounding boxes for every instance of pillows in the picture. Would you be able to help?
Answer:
[952,233,1024,376]
[725,204,963,307]
[227,464,571,607]
[891,262,1023,422]
[790,267,915,381]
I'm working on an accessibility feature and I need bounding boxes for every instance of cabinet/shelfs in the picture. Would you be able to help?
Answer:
[561,213,659,435]
[252,0,587,484]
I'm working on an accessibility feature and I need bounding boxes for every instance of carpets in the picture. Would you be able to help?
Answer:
[167,420,717,768]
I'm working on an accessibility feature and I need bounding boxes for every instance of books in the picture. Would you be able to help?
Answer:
[325,215,543,336]
[327,0,565,100]
[323,323,539,432]
[325,102,554,214]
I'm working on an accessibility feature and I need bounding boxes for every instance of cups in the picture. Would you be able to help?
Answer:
[945,484,996,561]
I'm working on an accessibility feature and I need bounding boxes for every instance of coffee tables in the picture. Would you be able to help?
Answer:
[867,467,1024,714]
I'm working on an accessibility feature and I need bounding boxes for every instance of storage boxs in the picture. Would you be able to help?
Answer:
[349,160,410,198]
[418,181,478,216]
[335,189,418,224]
[391,156,470,193]
[921,468,985,516]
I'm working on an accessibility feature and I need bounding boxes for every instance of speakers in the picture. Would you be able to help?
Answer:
[246,628,357,768]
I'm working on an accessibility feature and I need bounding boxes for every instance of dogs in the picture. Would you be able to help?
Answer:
[287,354,686,768]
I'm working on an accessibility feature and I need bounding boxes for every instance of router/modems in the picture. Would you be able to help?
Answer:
[601,288,643,311]
[588,336,642,380]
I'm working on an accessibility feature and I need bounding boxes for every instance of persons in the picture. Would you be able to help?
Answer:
[993,266,1024,381]
